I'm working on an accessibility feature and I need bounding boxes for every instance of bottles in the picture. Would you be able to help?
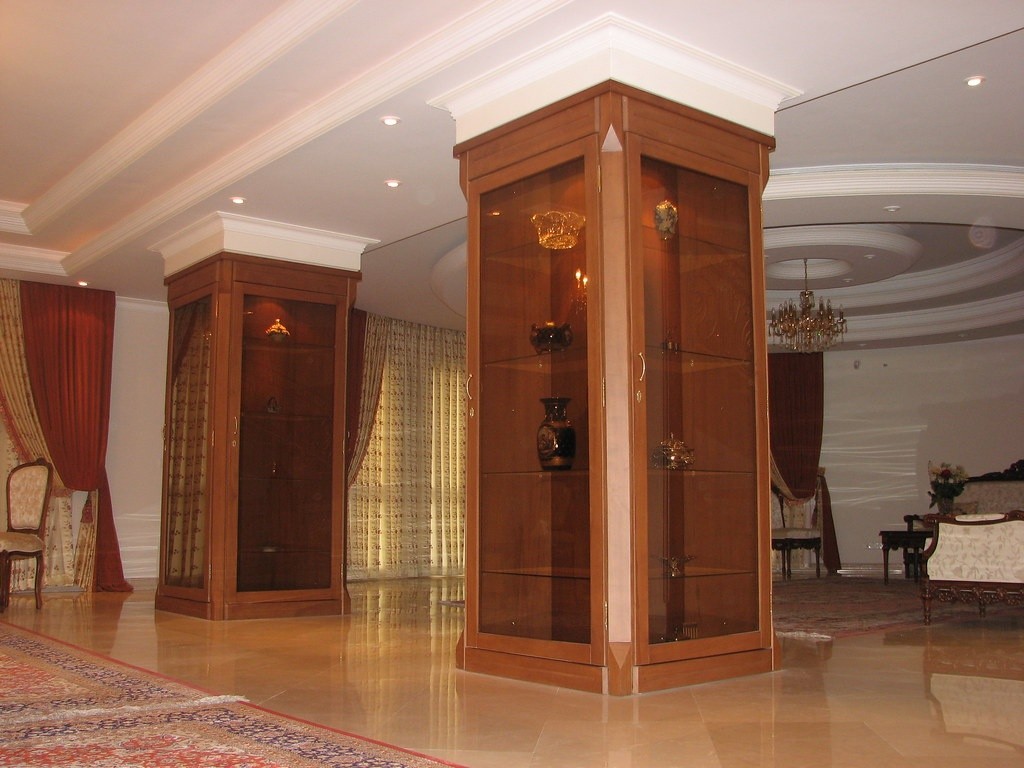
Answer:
[537,397,578,471]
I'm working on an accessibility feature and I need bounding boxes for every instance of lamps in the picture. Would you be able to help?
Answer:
[768,258,848,352]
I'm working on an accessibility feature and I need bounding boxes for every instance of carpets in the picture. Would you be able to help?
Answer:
[0,618,477,767]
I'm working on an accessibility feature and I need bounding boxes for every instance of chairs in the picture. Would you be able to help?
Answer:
[771,466,826,577]
[0,456,55,614]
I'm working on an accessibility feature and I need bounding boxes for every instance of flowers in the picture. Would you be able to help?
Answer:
[927,456,966,510]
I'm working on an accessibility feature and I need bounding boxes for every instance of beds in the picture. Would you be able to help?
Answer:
[918,459,1024,626]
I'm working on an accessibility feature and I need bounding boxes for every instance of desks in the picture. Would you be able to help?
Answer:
[879,531,933,584]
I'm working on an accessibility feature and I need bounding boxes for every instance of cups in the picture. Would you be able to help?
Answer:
[529,320,574,353]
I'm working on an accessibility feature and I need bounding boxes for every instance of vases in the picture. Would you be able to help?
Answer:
[936,496,954,514]
